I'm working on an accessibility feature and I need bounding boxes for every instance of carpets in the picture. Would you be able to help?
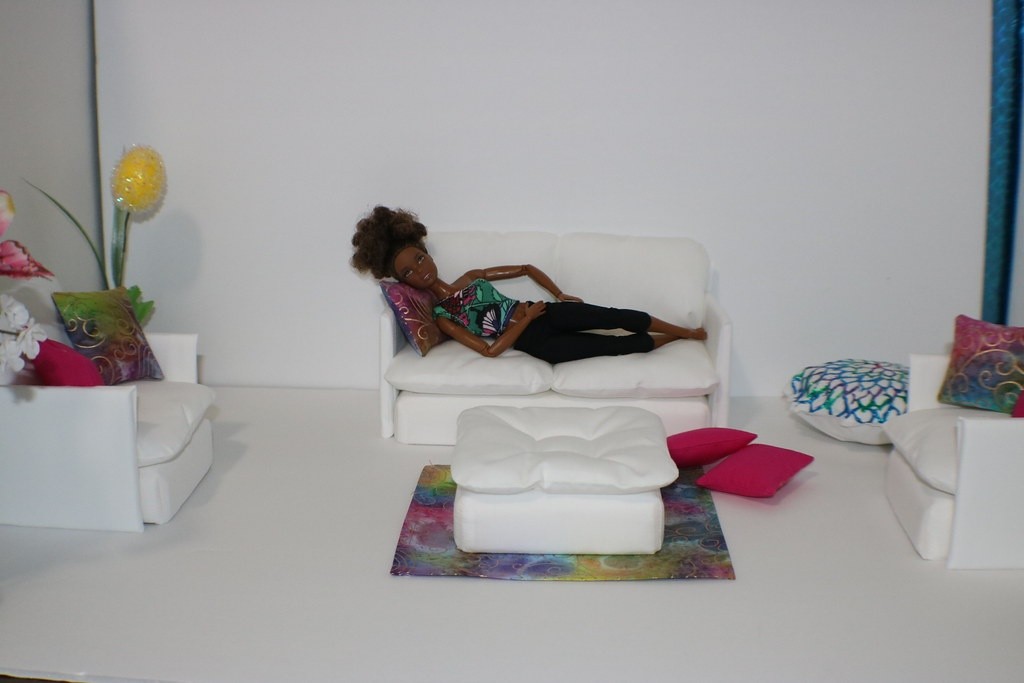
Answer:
[392,464,736,582]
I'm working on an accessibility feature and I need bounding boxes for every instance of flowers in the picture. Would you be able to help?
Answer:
[2,143,168,339]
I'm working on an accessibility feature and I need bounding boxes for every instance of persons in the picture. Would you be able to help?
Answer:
[351,205,708,366]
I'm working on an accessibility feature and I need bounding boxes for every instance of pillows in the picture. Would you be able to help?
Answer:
[696,444,815,498]
[667,427,759,467]
[51,287,166,385]
[789,356,911,450]
[24,337,106,386]
[936,314,1024,417]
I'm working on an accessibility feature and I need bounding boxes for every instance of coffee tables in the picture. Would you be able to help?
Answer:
[448,405,685,554]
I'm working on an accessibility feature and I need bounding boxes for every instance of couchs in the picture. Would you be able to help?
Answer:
[374,231,734,451]
[886,355,1024,569]
[0,331,216,533]
